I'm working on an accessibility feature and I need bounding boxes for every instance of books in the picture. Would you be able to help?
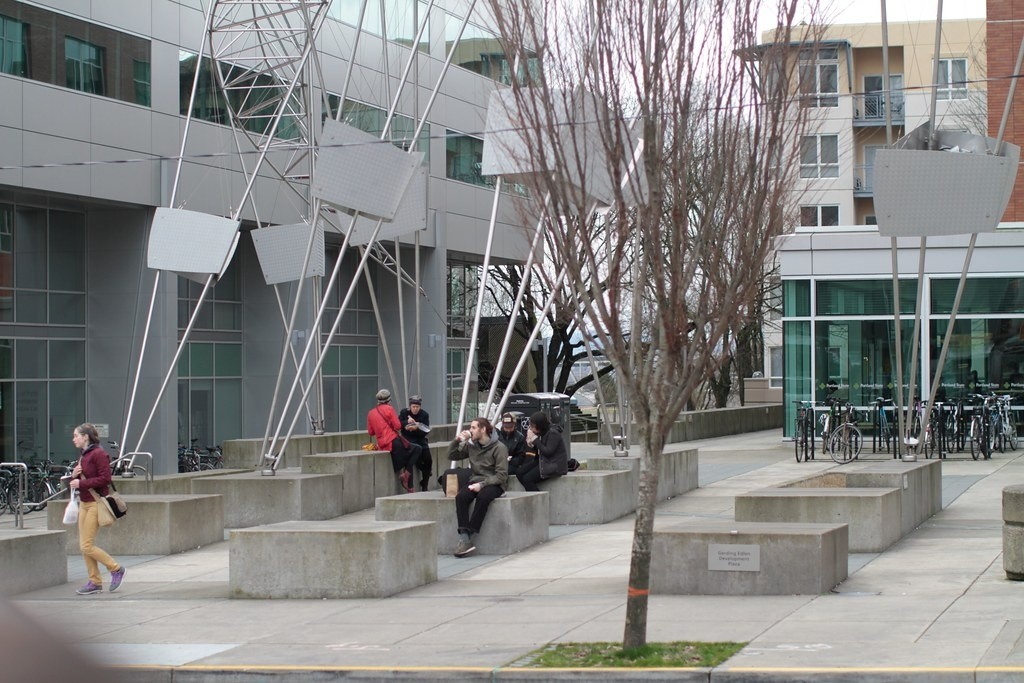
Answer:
[61,475,74,492]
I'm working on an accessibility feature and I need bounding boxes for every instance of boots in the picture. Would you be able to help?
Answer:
[399,470,411,490]
[408,487,415,493]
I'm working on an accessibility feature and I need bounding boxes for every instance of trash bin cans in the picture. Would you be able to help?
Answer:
[502,391,570,464]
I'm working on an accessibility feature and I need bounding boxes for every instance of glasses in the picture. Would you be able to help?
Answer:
[528,425,532,429]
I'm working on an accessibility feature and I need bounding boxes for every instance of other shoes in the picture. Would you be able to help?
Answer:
[420,463,432,477]
[419,480,428,491]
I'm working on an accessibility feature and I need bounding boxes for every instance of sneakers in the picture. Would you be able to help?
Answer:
[454,539,476,558]
[108,566,127,592]
[75,579,103,595]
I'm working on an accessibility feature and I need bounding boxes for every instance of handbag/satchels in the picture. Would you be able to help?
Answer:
[399,435,412,451]
[97,491,128,528]
[446,473,460,498]
[62,485,79,525]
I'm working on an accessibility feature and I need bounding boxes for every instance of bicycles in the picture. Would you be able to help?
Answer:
[829,403,865,465]
[867,391,1018,461]
[820,396,848,454]
[178,437,225,473]
[0,440,137,516]
[790,398,824,463]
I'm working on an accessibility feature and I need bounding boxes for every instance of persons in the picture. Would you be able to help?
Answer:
[495,413,525,475]
[367,390,422,489]
[1007,360,1024,401]
[515,411,568,492]
[398,395,433,493]
[68,423,127,595]
[447,418,509,557]
[964,369,982,393]
[826,380,842,399]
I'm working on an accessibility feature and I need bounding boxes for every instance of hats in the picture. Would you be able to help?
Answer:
[376,389,392,403]
[502,412,514,428]
[409,395,422,406]
